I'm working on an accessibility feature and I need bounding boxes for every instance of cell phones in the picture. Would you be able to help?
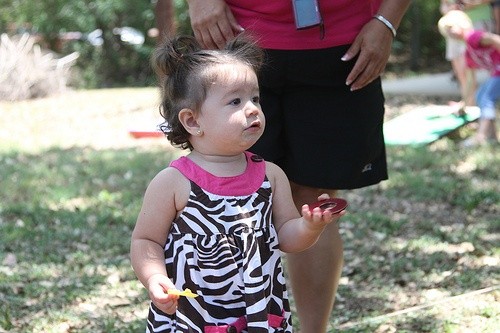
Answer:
[292,0,322,29]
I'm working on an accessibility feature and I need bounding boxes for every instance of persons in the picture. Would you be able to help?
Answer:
[437,0,500,148]
[130,34,345,333]
[186,0,410,333]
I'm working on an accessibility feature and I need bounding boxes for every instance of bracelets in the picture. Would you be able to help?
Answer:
[373,15,397,38]
